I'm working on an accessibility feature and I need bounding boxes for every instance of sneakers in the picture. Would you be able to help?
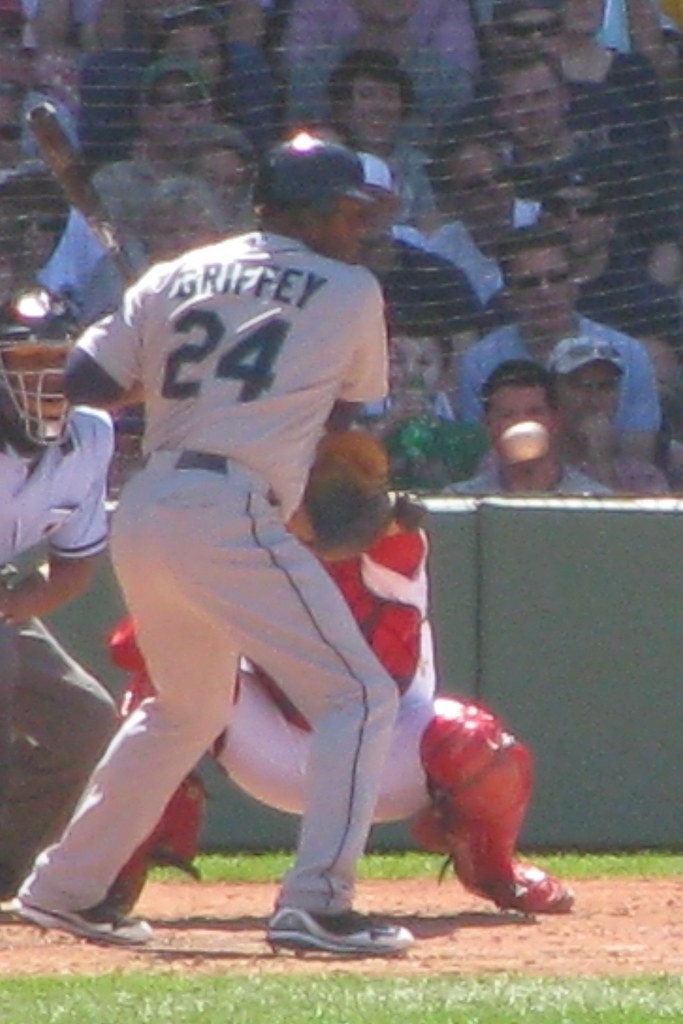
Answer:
[265,906,414,956]
[12,894,152,945]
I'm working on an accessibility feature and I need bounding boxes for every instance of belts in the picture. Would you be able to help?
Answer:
[136,450,282,507]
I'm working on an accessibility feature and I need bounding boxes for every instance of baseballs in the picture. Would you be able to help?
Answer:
[496,419,551,463]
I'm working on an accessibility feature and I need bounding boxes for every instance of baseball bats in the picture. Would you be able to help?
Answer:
[24,99,143,290]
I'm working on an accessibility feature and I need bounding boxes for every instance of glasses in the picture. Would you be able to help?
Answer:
[145,94,204,108]
[508,270,568,289]
[500,18,562,36]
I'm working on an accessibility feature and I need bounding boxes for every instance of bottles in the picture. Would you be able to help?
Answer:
[399,375,434,452]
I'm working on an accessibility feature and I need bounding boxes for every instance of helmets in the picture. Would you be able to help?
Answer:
[255,133,375,208]
[1,287,86,343]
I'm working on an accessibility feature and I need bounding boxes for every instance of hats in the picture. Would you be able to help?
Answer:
[152,4,222,47]
[549,337,626,373]
[140,55,212,101]
[357,151,394,196]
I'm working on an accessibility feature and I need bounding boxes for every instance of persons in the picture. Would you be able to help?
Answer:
[10,140,415,959]
[108,501,574,914]
[0,0,683,497]
[0,282,124,895]
